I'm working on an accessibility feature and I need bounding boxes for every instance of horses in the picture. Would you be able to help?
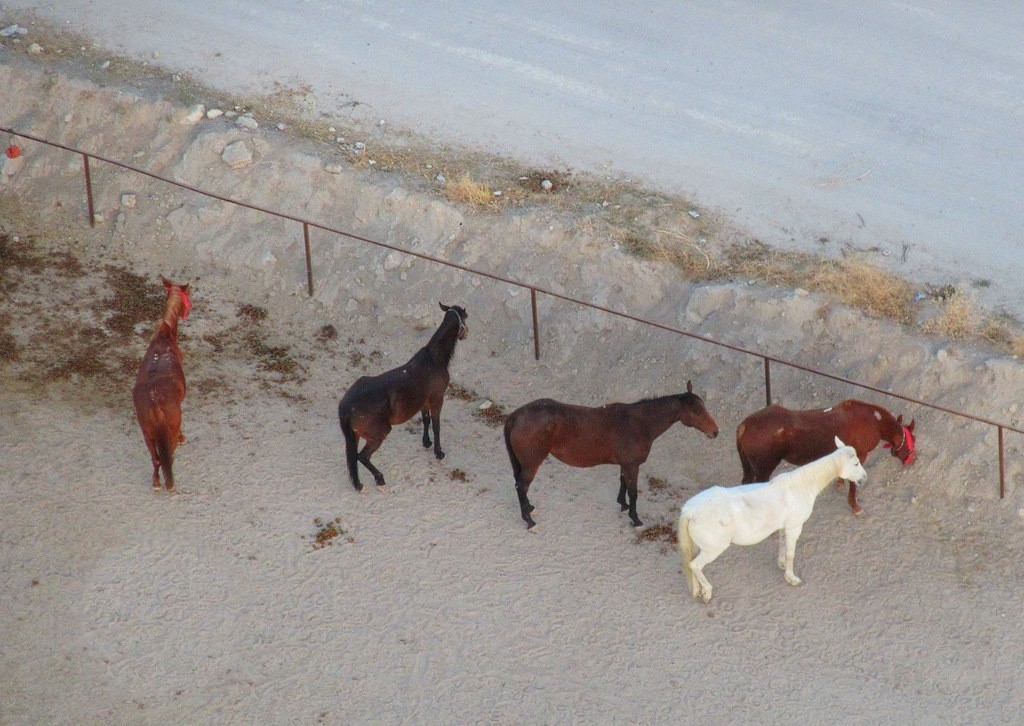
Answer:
[338,302,469,490]
[133,274,191,488]
[736,400,918,518]
[504,379,719,528]
[679,436,869,604]
